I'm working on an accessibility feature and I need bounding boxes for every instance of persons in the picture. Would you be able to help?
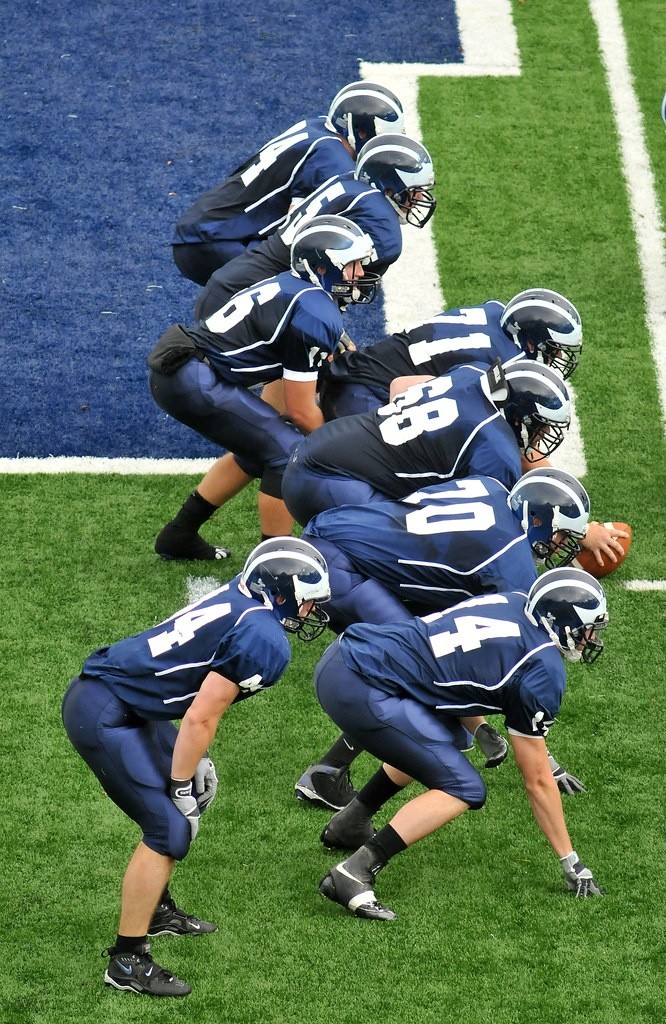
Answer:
[281,360,567,530]
[173,82,404,289]
[323,287,582,422]
[316,567,612,921]
[62,536,331,997]
[195,134,436,330]
[147,215,381,562]
[295,467,590,810]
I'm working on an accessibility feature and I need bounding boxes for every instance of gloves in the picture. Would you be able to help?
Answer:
[545,748,588,795]
[475,723,509,768]
[194,752,218,809]
[168,777,200,841]
[560,851,604,899]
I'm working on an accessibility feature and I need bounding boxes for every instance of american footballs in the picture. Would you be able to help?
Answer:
[567,521,633,580]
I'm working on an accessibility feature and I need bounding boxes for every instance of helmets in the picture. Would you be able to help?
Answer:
[479,356,572,462]
[507,468,591,570]
[354,134,437,229]
[289,214,380,313]
[238,536,331,642]
[324,81,407,158]
[523,566,610,665]
[500,288,582,381]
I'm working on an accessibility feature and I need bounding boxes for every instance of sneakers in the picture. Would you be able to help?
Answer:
[295,765,358,811]
[320,797,377,851]
[101,944,190,996]
[147,892,217,938]
[155,522,231,560]
[319,845,399,921]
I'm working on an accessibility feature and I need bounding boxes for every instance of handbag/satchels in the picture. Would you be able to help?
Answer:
[148,322,215,375]
